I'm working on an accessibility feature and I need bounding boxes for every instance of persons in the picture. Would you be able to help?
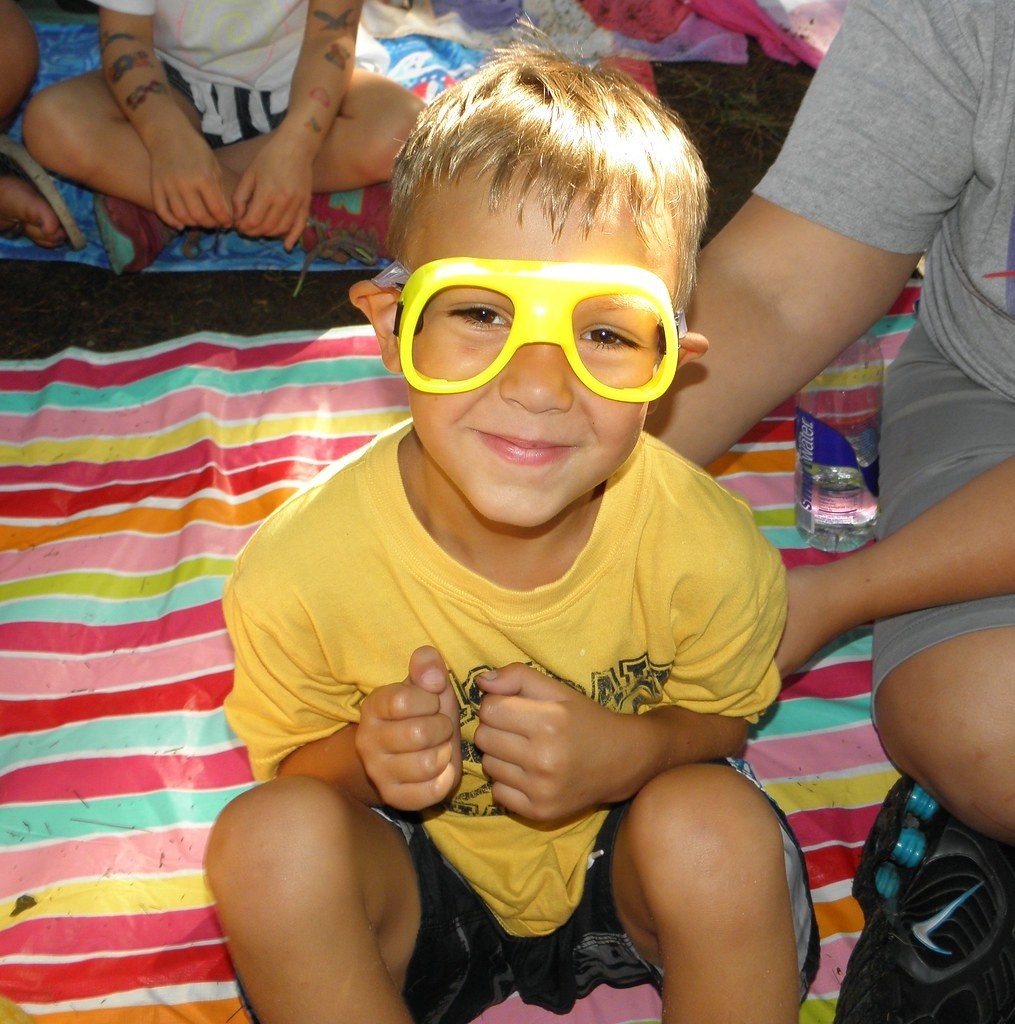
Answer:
[196,32,824,1024]
[641,1,1015,852]
[0,0,428,251]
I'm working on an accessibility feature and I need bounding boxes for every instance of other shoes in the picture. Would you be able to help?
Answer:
[300,182,394,265]
[96,193,173,272]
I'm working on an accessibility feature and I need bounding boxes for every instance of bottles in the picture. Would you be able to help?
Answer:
[795,329,882,554]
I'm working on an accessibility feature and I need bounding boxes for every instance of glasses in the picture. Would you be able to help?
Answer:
[392,257,681,405]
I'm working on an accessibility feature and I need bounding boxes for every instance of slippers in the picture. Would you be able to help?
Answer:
[0,134,87,251]
